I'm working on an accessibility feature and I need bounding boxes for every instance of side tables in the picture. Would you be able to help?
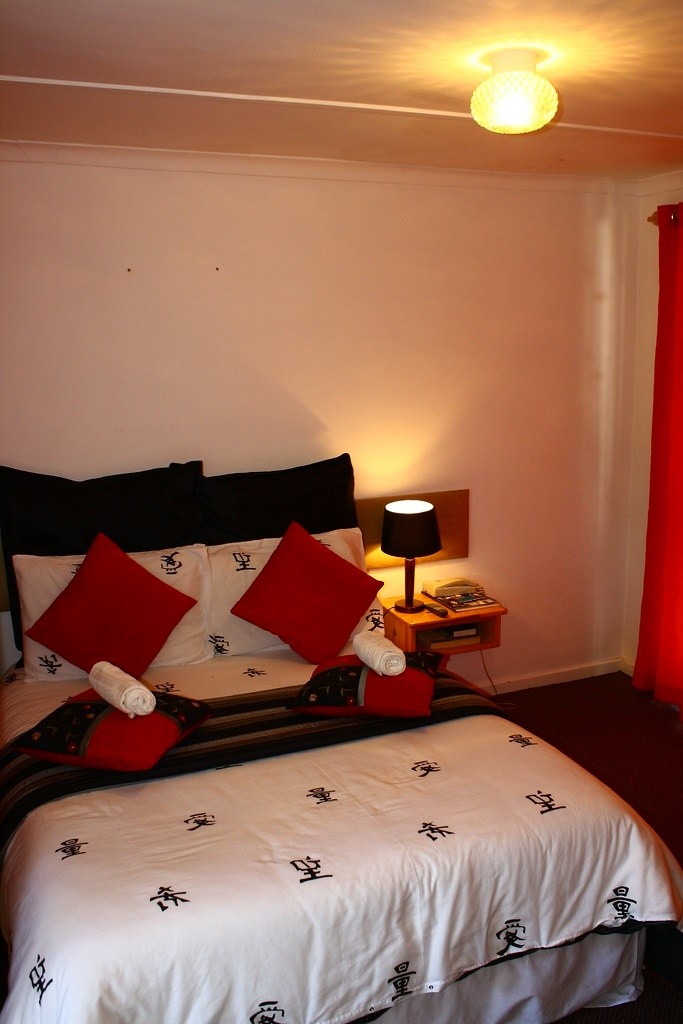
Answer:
[379,591,508,663]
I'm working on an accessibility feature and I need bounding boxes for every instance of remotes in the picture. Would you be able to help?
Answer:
[424,602,449,618]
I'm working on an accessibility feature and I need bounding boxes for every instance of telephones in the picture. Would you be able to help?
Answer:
[423,577,476,598]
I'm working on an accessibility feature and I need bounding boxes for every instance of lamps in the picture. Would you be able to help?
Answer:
[380,498,443,615]
[469,47,562,138]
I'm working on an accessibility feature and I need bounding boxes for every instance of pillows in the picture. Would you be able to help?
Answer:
[10,542,218,682]
[1,458,206,651]
[25,682,216,773]
[169,453,361,546]
[207,527,389,659]
[24,531,199,684]
[288,648,447,716]
[230,519,385,663]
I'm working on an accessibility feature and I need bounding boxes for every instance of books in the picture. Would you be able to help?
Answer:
[416,623,480,649]
[421,590,499,612]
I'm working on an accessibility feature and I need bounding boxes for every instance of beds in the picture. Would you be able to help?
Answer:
[1,641,682,1024]
